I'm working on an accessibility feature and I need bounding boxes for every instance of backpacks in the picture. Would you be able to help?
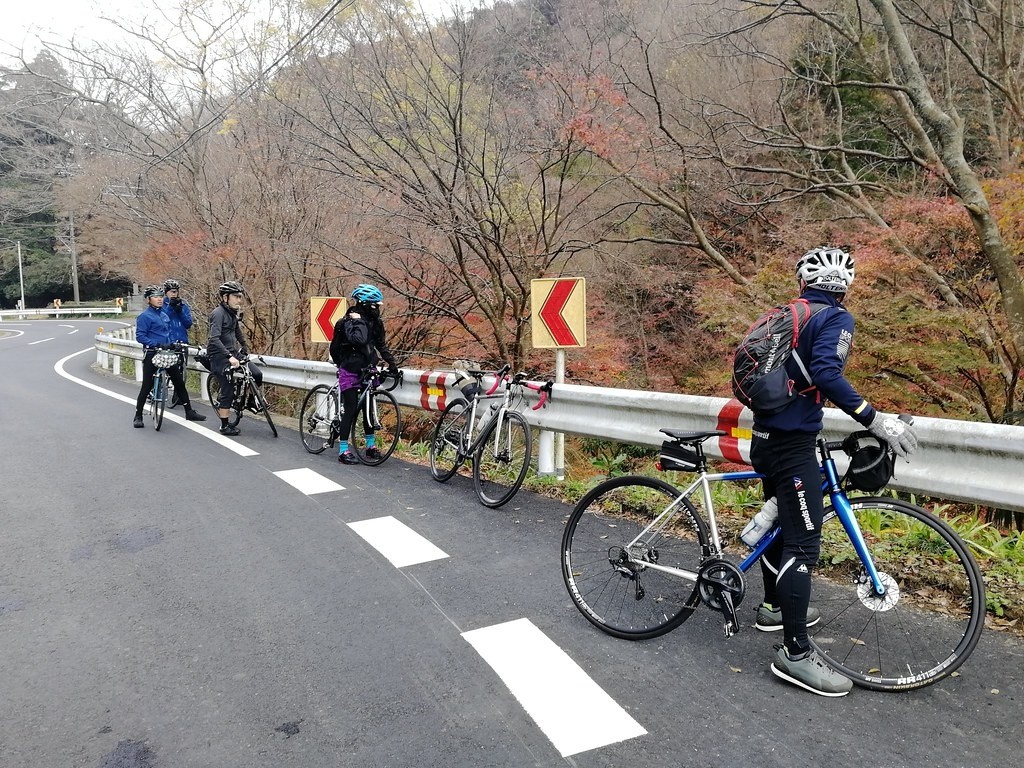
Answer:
[730,298,842,413]
[329,315,372,364]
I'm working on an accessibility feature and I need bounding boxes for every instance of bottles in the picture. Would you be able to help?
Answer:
[235,377,243,397]
[476,402,499,433]
[739,493,778,547]
[341,392,345,417]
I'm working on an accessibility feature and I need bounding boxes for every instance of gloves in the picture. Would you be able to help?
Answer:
[170,298,183,312]
[868,412,918,458]
[157,342,175,351]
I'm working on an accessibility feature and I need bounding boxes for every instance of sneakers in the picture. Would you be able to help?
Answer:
[367,447,383,461]
[755,601,822,632]
[769,644,854,699]
[219,424,237,436]
[246,399,269,408]
[185,410,206,420]
[133,414,144,427]
[338,451,359,466]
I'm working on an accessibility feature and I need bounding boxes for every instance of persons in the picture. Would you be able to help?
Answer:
[749,245,918,696]
[133,285,206,422]
[207,281,270,434]
[161,279,192,405]
[331,283,398,465]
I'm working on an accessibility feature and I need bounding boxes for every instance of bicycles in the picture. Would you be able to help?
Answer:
[430,364,554,509]
[560,412,988,693]
[206,348,279,438]
[143,339,191,431]
[299,362,403,466]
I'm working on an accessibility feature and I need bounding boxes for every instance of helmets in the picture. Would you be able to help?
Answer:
[144,286,164,298]
[794,246,855,293]
[219,280,243,295]
[163,280,180,292]
[352,284,383,302]
